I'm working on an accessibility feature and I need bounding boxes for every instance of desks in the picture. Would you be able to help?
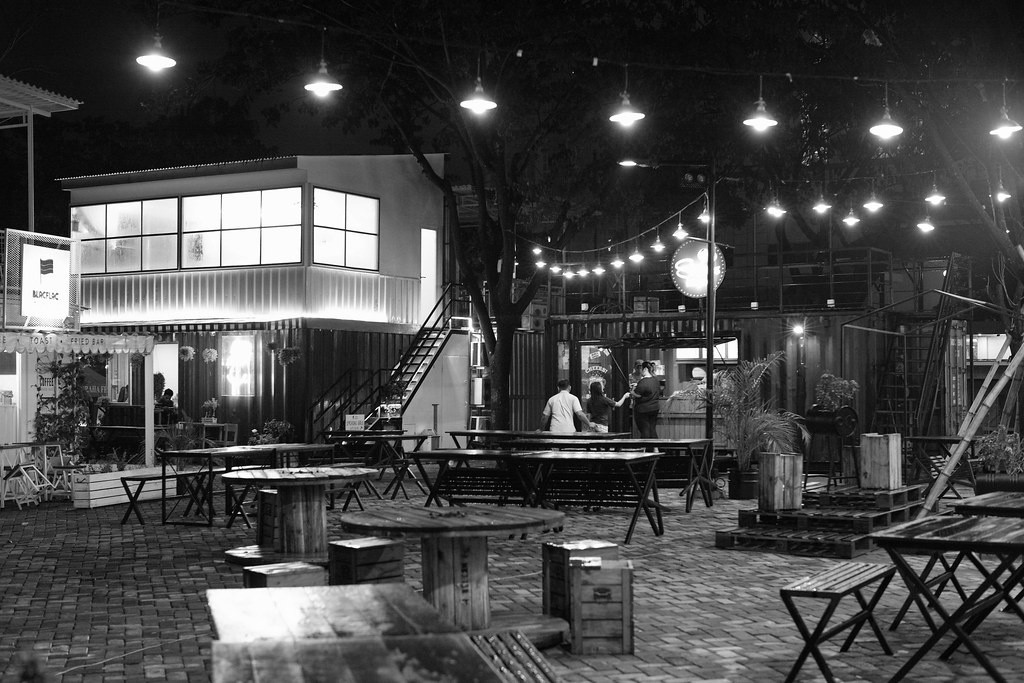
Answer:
[88,426,169,447]
[0,441,68,508]
[869,490,1024,683]
[205,582,507,683]
[179,422,225,449]
[368,417,403,447]
[904,436,979,485]
[160,429,714,631]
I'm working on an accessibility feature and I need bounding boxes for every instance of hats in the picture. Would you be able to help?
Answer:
[635,359,644,366]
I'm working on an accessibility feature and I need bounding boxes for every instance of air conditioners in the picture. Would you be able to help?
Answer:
[521,304,548,330]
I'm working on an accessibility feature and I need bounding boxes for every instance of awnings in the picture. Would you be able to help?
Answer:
[598,337,736,381]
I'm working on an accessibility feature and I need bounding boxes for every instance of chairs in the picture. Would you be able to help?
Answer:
[215,423,238,447]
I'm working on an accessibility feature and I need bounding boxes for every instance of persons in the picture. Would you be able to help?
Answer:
[631,361,661,453]
[586,382,631,433]
[536,379,596,433]
[158,389,173,407]
[629,359,644,383]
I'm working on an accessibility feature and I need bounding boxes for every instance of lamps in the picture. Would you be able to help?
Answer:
[678,171,709,189]
[460,52,497,114]
[135,4,177,72]
[303,29,343,96]
[62,316,75,329]
[743,75,778,130]
[764,165,1011,233]
[869,84,903,137]
[989,81,1023,140]
[609,65,645,127]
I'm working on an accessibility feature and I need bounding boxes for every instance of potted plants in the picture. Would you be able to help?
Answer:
[975,424,1024,496]
[72,415,218,507]
[806,373,860,437]
[661,351,812,500]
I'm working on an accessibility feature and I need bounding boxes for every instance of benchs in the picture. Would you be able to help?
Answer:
[383,459,438,501]
[226,481,364,529]
[318,463,383,502]
[120,464,273,525]
[662,446,736,470]
[471,630,565,683]
[780,562,898,683]
[947,457,985,500]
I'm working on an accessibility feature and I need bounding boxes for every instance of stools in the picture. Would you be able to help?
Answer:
[50,464,86,501]
[243,561,328,589]
[329,537,406,586]
[3,463,55,510]
[257,489,277,545]
[543,539,635,656]
